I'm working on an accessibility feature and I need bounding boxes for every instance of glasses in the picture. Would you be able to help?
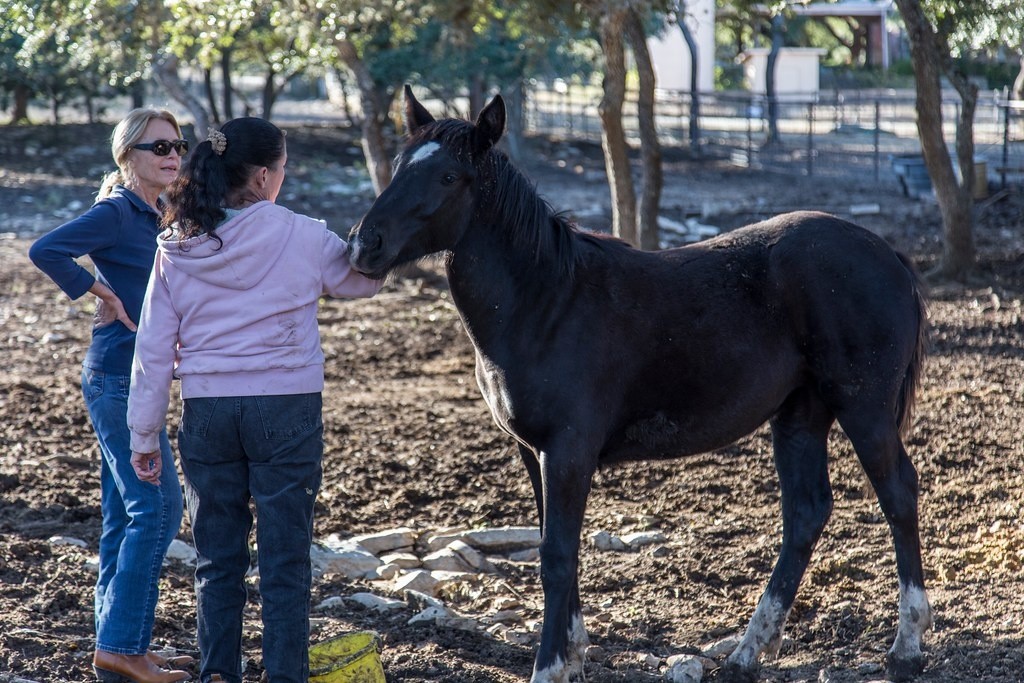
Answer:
[132,139,190,156]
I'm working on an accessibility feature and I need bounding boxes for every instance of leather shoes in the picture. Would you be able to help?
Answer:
[91,649,195,683]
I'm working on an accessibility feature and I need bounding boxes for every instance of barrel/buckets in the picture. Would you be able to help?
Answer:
[308,632,386,683]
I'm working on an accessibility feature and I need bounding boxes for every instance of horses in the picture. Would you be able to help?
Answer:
[346,83,937,683]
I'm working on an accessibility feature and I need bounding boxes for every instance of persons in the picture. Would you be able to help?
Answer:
[28,108,185,683]
[125,117,387,683]
